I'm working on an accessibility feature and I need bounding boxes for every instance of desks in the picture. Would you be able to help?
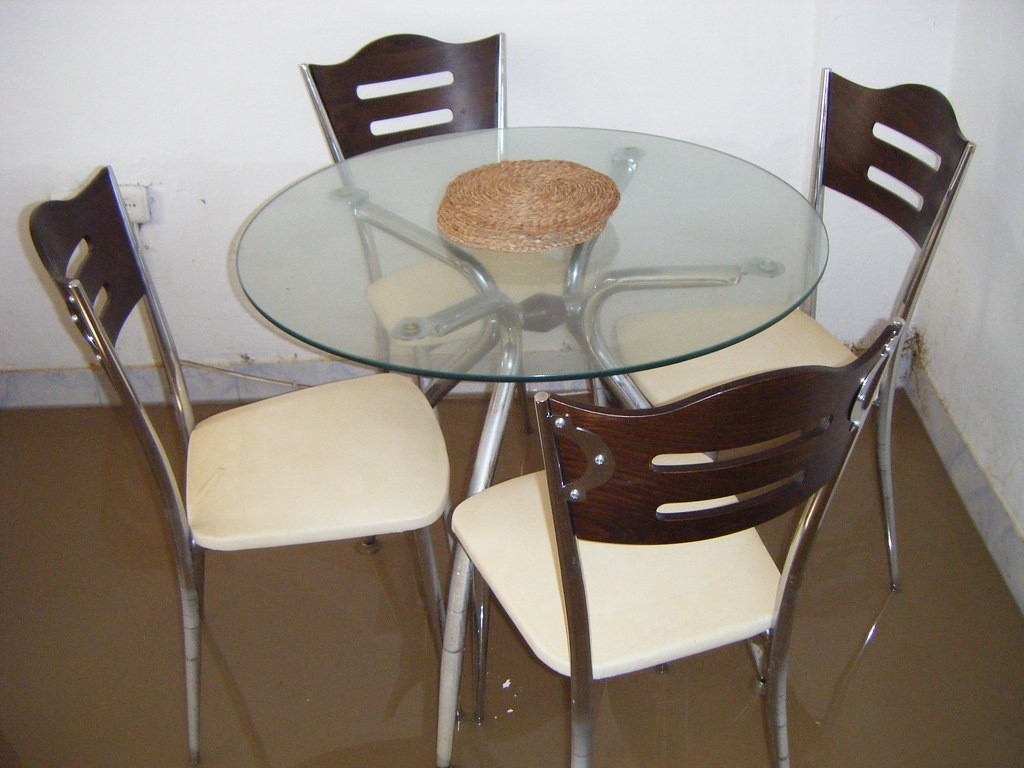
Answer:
[234,124,830,768]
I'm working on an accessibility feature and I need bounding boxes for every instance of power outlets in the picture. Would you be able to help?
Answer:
[117,185,151,225]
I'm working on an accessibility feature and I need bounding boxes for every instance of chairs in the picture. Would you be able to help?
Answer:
[15,165,467,768]
[577,67,978,594]
[296,30,593,432]
[451,317,905,768]
